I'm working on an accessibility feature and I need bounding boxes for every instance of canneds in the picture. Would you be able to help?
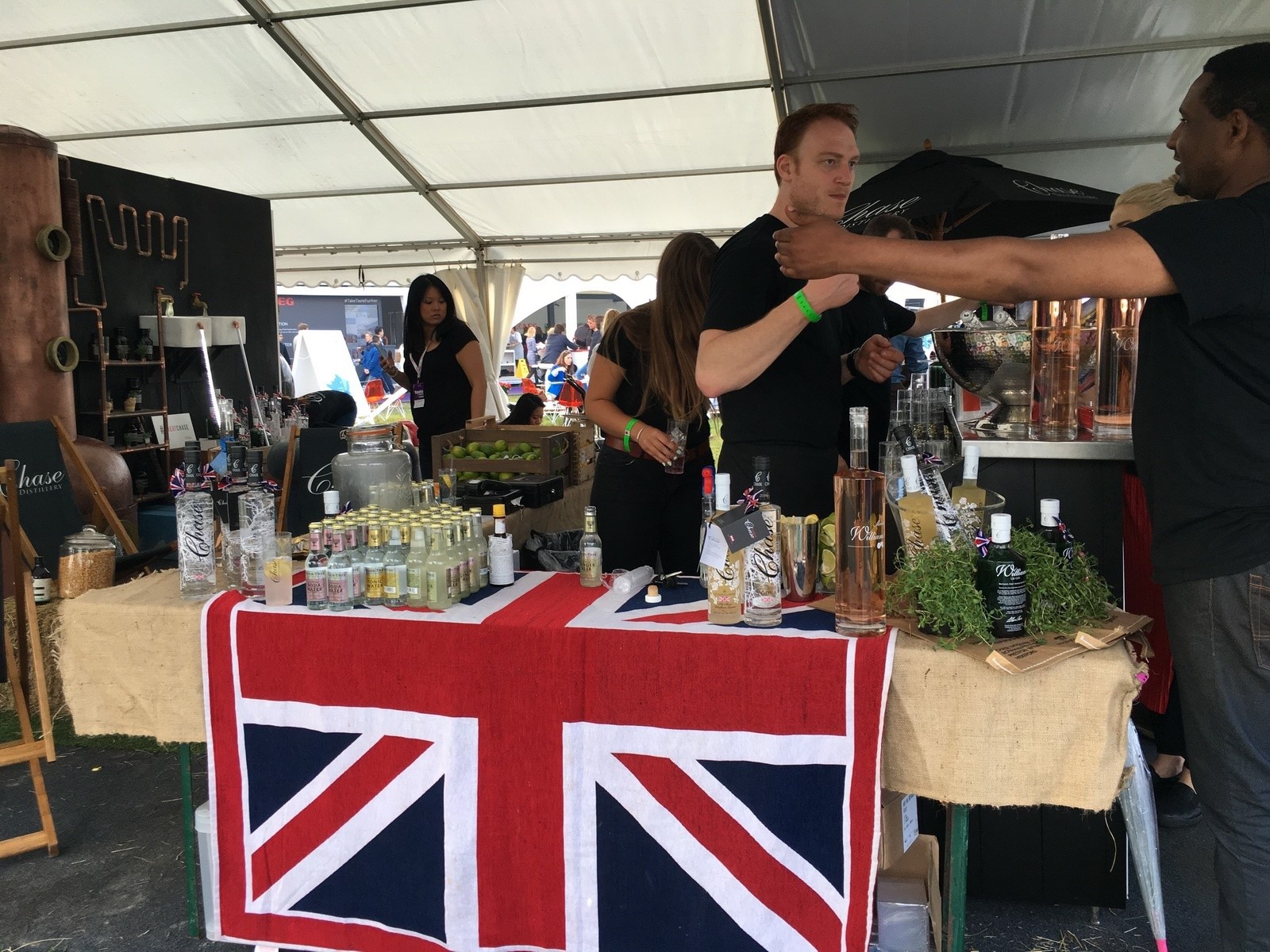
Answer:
[960,310,983,328]
[992,311,1018,327]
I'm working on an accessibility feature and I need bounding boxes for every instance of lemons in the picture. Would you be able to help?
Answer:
[780,514,818,524]
[819,512,839,591]
[443,440,563,481]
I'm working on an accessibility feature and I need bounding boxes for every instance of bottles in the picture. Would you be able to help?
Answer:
[57,524,116,600]
[1039,498,1074,570]
[215,385,309,448]
[1092,298,1144,442]
[490,504,514,588]
[706,472,742,625]
[304,479,489,612]
[743,457,782,627]
[32,556,52,606]
[833,406,887,638]
[975,512,1027,638]
[580,505,603,587]
[960,304,1019,328]
[330,424,413,513]
[1027,232,1082,442]
[175,441,275,602]
[891,423,986,567]
[98,326,153,449]
[700,469,715,589]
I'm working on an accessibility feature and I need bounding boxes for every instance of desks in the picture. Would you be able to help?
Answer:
[57,555,1150,952]
[946,406,1134,460]
[570,349,589,370]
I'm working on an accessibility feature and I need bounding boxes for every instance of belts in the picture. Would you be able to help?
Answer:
[605,434,711,461]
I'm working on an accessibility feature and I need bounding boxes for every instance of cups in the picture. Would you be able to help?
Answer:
[665,418,688,474]
[437,467,457,507]
[879,373,952,476]
[782,516,821,602]
[262,530,293,606]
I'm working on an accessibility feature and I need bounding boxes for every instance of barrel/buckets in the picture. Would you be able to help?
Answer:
[885,477,1006,557]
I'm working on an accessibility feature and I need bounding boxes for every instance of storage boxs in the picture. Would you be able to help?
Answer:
[877,835,942,952]
[431,415,596,515]
[876,786,919,871]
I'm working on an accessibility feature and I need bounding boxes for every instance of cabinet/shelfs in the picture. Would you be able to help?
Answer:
[501,350,516,378]
[79,359,174,503]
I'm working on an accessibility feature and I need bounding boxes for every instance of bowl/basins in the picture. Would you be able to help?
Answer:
[930,326,1032,433]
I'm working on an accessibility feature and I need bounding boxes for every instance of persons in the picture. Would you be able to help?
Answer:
[840,217,921,481]
[771,40,1270,952]
[585,232,716,585]
[279,323,309,369]
[378,274,487,482]
[1107,173,1198,810]
[499,304,621,429]
[693,100,906,535]
[350,327,405,396]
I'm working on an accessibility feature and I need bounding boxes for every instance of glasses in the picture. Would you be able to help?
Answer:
[587,322,595,325]
[564,350,570,354]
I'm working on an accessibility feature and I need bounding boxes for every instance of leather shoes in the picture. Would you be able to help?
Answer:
[1156,783,1204,827]
[1148,764,1184,797]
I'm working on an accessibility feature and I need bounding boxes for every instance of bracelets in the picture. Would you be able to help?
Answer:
[846,347,862,377]
[387,369,398,377]
[637,426,651,441]
[793,289,823,323]
[623,419,638,453]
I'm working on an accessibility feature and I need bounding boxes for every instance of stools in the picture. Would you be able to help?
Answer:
[526,363,553,388]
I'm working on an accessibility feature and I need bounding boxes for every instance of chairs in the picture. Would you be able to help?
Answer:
[0,416,151,858]
[521,378,584,426]
[276,423,403,558]
[365,379,406,421]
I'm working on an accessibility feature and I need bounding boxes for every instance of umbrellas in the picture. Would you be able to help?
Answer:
[844,140,1123,304]
